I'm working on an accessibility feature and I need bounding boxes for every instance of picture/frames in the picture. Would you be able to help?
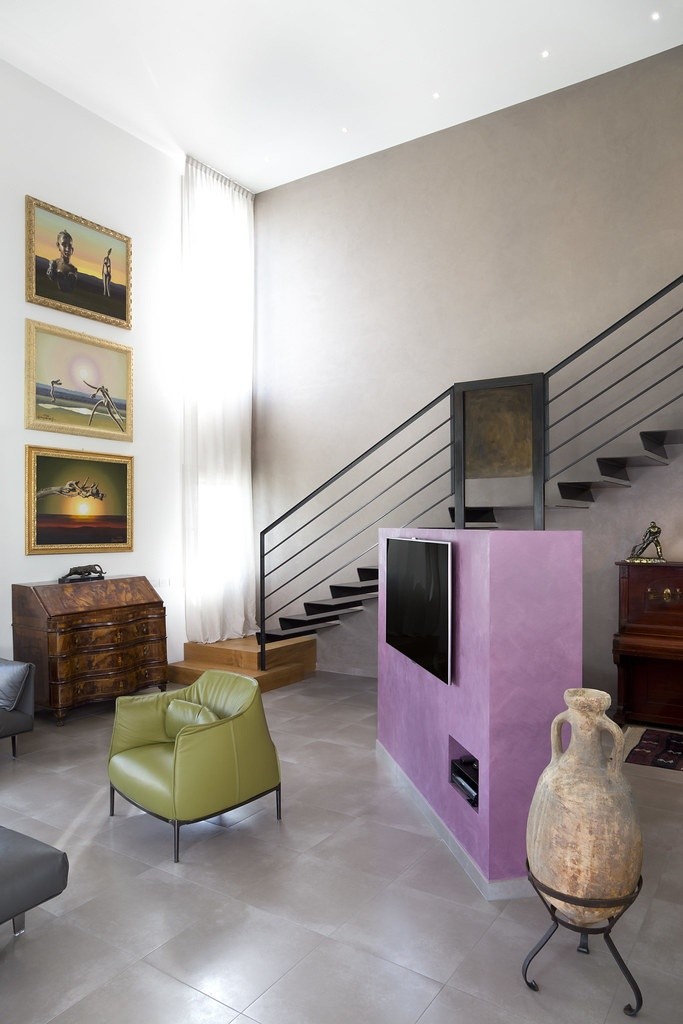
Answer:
[24,194,134,332]
[24,444,136,557]
[24,318,135,444]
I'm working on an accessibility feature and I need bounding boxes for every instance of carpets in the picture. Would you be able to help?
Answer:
[609,725,683,788]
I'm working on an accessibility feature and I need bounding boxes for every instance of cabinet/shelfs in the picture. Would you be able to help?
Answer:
[11,576,169,724]
[610,558,683,735]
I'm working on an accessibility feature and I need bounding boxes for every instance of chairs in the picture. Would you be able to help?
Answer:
[0,658,36,760]
[0,825,70,937]
[108,669,285,864]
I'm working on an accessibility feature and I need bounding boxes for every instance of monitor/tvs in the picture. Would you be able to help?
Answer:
[385,537,453,686]
